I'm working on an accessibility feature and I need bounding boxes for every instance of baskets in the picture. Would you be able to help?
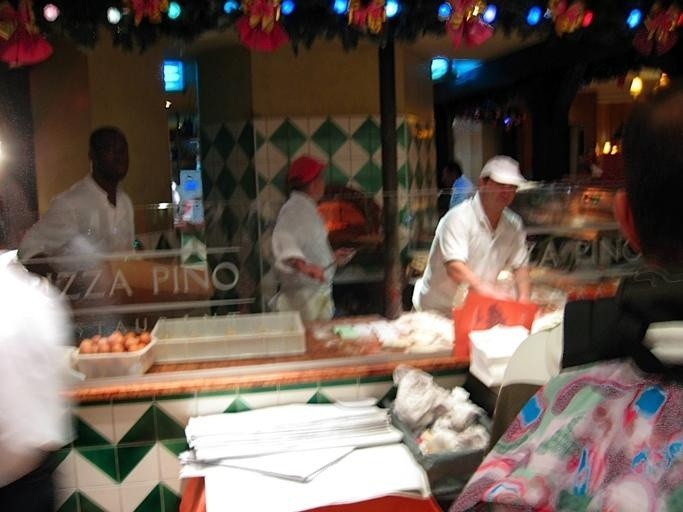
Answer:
[76,336,156,376]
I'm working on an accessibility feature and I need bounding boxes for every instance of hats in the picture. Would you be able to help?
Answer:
[288,156,321,183]
[480,155,528,186]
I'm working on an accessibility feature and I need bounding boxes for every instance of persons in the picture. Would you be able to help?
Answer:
[407,152,536,318]
[484,73,682,457]
[268,150,357,323]
[441,161,474,212]
[16,122,144,336]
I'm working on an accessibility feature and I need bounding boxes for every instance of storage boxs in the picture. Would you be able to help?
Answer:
[70,338,158,375]
[151,310,306,365]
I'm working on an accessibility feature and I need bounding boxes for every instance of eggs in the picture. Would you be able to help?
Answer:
[79,332,151,352]
